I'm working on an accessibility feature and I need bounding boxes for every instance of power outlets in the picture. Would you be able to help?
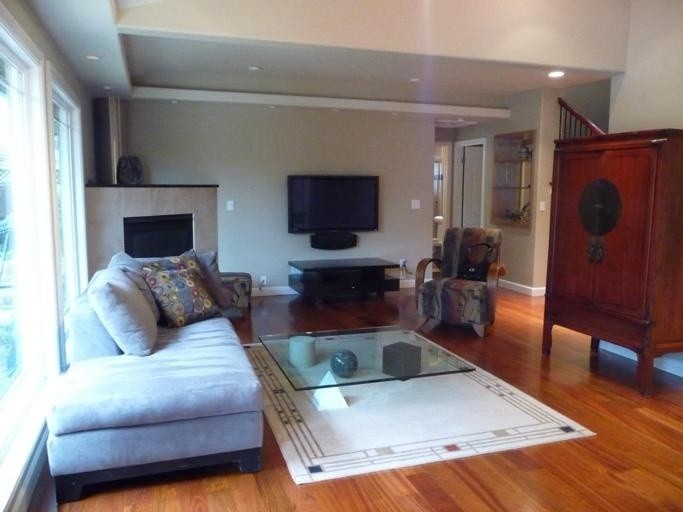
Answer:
[260,276,267,286]
[400,259,408,268]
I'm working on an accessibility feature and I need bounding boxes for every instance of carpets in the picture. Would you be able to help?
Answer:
[242,326,598,485]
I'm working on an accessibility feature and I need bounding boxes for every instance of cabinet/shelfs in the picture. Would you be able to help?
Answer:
[286,257,404,303]
[540,127,683,398]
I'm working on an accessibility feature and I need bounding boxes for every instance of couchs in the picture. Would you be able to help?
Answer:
[48,268,269,504]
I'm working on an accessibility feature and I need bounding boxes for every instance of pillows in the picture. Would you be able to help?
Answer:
[83,250,235,358]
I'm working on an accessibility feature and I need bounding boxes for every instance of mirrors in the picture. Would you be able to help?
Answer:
[490,129,537,232]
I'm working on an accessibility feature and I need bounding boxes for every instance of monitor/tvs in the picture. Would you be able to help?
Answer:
[287,175,379,233]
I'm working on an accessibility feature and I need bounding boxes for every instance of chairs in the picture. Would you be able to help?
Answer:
[414,226,507,339]
